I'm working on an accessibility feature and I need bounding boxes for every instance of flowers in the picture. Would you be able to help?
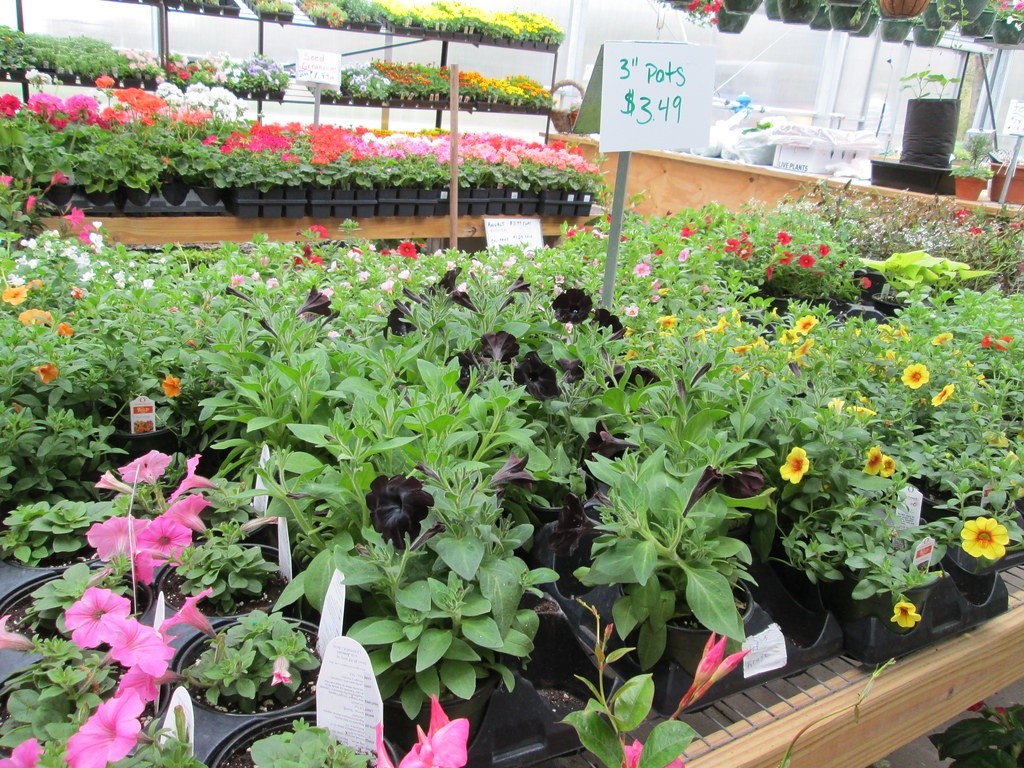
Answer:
[251,0,295,14]
[686,0,725,28]
[0,25,293,93]
[0,176,1024,768]
[182,0,201,7]
[372,0,569,45]
[989,0,1024,25]
[321,57,559,110]
[0,70,609,195]
[298,0,377,29]
[205,0,220,6]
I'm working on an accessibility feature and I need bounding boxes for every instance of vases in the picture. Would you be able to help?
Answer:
[378,16,561,54]
[109,418,185,466]
[385,683,495,754]
[665,580,758,681]
[848,574,936,636]
[871,157,953,195]
[296,0,382,33]
[991,162,1024,206]
[155,539,303,620]
[0,68,286,102]
[163,0,201,14]
[173,614,325,713]
[41,186,593,218]
[856,274,886,303]
[943,545,995,606]
[242,0,294,23]
[205,712,401,768]
[203,0,241,16]
[670,0,1024,47]
[322,96,553,115]
[0,568,155,649]
[871,292,910,317]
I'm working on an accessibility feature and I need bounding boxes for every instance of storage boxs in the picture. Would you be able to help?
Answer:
[771,131,878,180]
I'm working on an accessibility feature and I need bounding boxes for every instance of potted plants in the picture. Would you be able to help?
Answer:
[897,68,965,170]
[950,131,995,204]
